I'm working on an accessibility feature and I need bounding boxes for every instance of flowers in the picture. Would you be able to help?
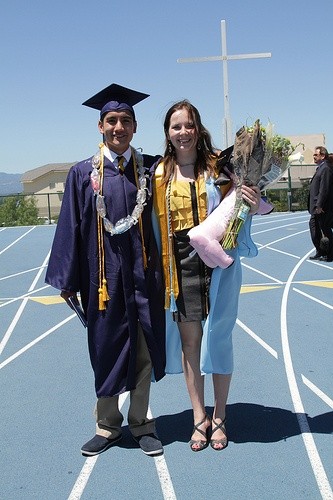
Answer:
[222,110,304,250]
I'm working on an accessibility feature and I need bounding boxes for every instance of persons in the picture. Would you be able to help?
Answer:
[150,100,261,451]
[307,146,333,262]
[45,83,221,456]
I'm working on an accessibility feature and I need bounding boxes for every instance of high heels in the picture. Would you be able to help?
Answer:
[190,414,212,452]
[210,416,227,450]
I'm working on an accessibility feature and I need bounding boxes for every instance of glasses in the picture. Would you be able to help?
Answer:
[313,153,318,156]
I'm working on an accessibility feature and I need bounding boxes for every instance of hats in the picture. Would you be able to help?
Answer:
[82,83,151,122]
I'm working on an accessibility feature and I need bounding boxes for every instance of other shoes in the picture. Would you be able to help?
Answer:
[309,253,329,261]
[80,434,124,456]
[131,433,163,456]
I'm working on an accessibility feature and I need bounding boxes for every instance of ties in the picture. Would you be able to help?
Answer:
[316,167,319,171]
[116,156,125,173]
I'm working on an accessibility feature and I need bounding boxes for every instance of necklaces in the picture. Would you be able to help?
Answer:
[175,159,194,167]
